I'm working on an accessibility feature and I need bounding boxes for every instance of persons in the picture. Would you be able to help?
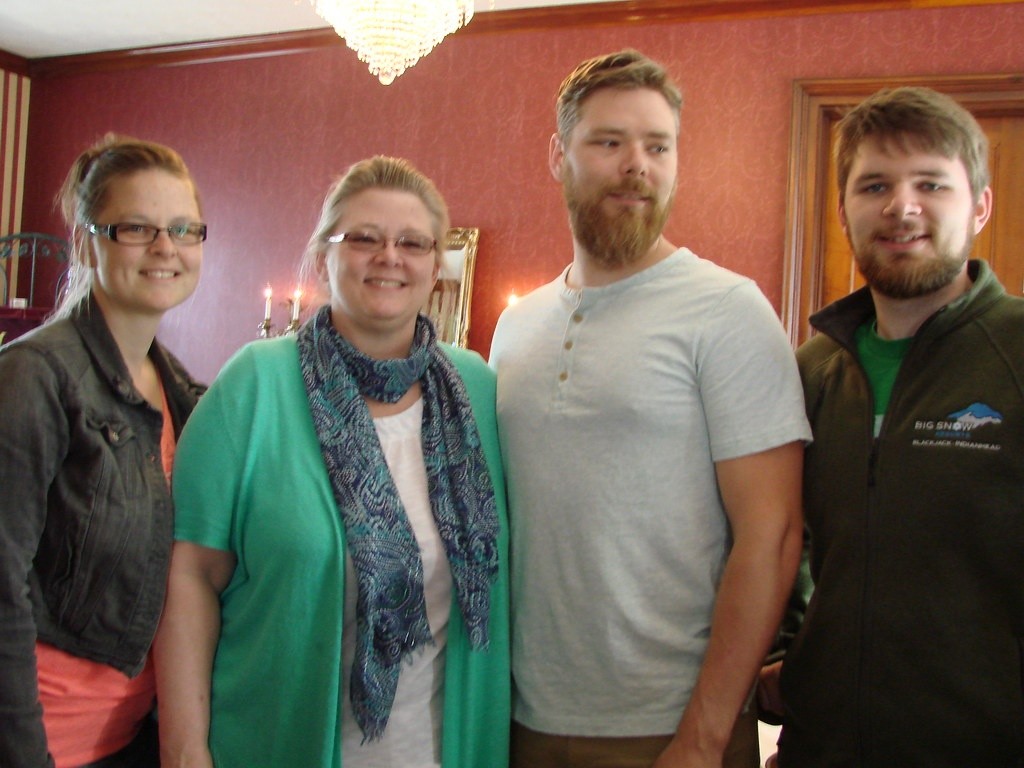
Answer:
[758,87,1024,768]
[154,155,512,768]
[488,51,813,768]
[1,136,209,768]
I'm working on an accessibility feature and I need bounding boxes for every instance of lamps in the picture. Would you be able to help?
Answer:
[311,0,482,85]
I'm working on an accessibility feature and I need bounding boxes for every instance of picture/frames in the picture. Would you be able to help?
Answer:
[418,226,479,353]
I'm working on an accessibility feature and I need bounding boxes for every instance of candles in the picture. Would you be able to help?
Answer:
[264,287,273,318]
[293,288,302,319]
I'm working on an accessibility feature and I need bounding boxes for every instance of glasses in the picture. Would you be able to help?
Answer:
[82,222,209,245]
[325,227,437,255]
